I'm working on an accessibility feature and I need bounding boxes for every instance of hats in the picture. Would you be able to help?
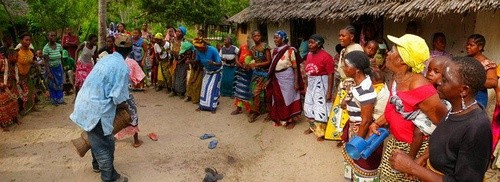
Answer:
[387,34,430,74]
[154,33,163,39]
[114,34,131,47]
[179,26,186,34]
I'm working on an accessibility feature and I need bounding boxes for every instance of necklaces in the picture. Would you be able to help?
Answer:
[449,100,477,114]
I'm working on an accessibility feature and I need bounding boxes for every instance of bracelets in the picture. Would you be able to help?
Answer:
[213,62,216,65]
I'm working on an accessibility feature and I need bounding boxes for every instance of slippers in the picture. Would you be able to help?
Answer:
[207,139,218,149]
[200,133,214,139]
[148,132,158,141]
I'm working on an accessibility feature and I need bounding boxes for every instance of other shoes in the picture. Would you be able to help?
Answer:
[102,174,128,182]
[93,169,99,173]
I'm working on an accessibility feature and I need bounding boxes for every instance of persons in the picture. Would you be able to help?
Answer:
[369,34,448,182]
[100,22,222,115]
[0,31,46,131]
[114,56,146,148]
[69,34,133,182]
[263,31,305,128]
[63,50,75,95]
[43,31,64,105]
[219,37,239,99]
[324,25,391,148]
[73,34,97,104]
[61,27,80,58]
[248,29,272,123]
[389,56,493,182]
[341,51,384,182]
[231,33,255,115]
[303,34,334,142]
[424,33,500,171]
